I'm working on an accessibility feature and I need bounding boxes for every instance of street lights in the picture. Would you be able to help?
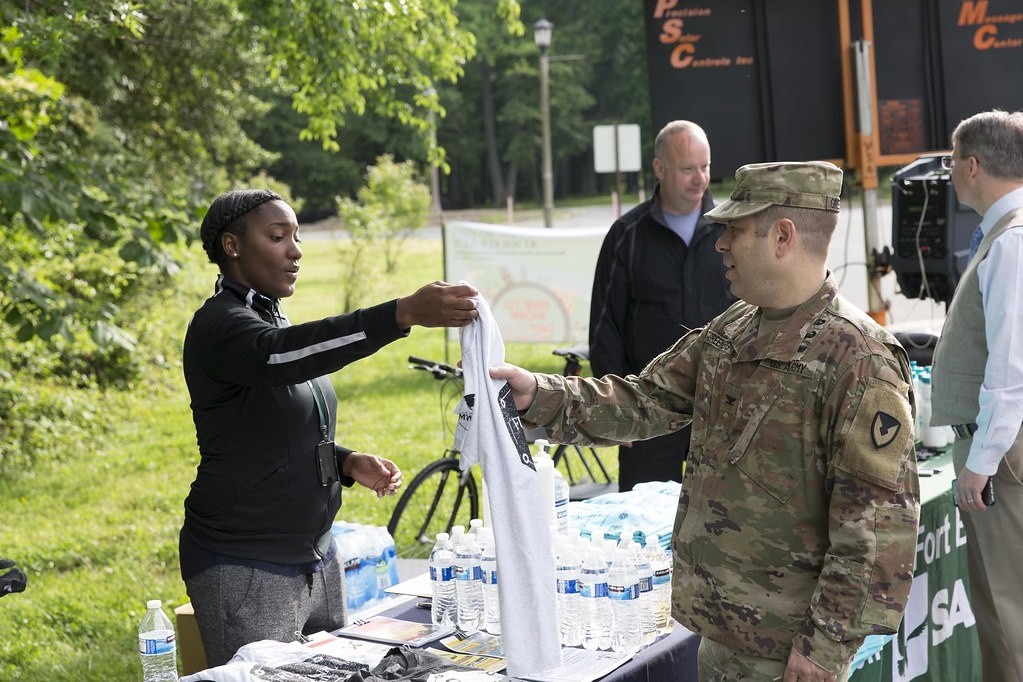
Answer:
[534,18,557,229]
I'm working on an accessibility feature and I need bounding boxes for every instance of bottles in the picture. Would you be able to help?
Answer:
[331,519,399,616]
[910,360,955,448]
[138,599,179,682]
[428,481,683,660]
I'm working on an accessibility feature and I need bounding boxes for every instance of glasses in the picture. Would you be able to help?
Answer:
[941,156,979,171]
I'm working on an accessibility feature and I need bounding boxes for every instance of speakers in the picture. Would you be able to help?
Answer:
[887,151,984,302]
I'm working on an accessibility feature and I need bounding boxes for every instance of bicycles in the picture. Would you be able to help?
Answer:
[386,344,612,561]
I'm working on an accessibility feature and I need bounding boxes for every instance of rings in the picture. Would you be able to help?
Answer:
[967,500,973,503]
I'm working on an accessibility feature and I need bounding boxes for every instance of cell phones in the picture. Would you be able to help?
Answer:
[952,475,995,507]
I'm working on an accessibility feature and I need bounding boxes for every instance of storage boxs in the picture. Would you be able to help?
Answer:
[174,602,208,677]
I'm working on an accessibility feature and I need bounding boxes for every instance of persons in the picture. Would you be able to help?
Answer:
[178,189,479,669]
[929,110,1023,682]
[459,161,920,682]
[589,121,741,493]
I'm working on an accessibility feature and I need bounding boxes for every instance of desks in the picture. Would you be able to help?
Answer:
[306,446,982,682]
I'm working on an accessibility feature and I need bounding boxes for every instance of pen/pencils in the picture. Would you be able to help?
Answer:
[415,602,432,608]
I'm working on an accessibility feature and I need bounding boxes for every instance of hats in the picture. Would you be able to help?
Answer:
[703,161,844,224]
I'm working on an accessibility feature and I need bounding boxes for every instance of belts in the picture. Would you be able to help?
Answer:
[951,423,978,439]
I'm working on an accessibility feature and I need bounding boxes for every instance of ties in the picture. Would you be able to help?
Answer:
[968,227,983,260]
